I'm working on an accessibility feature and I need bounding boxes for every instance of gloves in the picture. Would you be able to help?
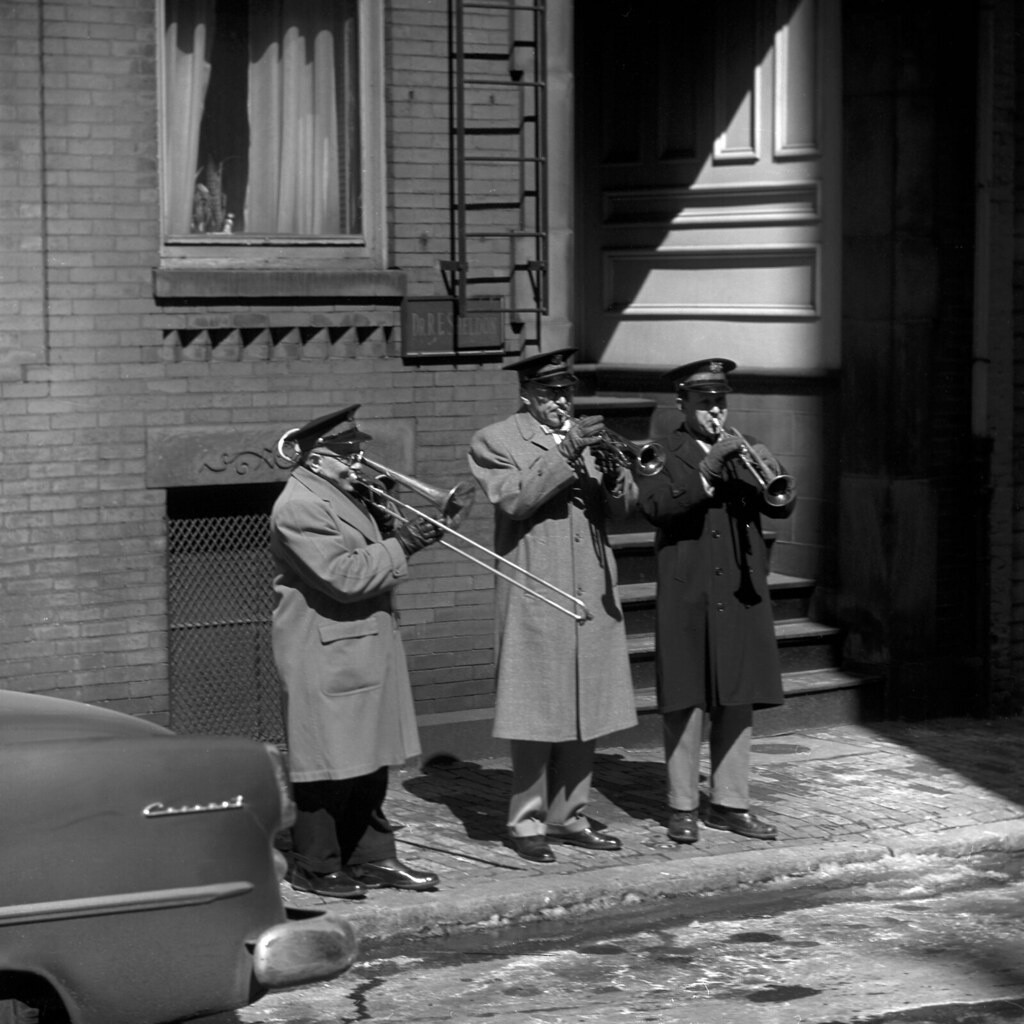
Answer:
[699,436,743,486]
[746,443,781,475]
[394,515,448,557]
[559,414,603,462]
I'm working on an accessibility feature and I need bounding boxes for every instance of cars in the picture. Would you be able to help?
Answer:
[1,686,359,1024]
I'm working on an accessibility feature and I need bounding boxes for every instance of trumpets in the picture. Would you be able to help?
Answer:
[555,406,669,477]
[711,417,800,507]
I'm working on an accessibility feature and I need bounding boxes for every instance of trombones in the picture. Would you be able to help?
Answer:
[275,426,591,624]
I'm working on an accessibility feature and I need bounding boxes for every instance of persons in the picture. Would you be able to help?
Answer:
[636,357,834,842]
[466,347,639,863]
[268,404,447,897]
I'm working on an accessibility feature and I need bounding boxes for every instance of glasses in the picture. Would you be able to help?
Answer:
[314,450,366,466]
[534,386,574,403]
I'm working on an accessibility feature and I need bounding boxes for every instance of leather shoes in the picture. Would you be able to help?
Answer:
[551,829,621,850]
[667,810,698,843]
[705,806,776,839]
[292,868,369,900]
[507,834,556,861]
[359,862,439,890]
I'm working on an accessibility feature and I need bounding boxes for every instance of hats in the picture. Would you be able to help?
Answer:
[284,403,373,453]
[502,348,579,388]
[662,358,736,397]
